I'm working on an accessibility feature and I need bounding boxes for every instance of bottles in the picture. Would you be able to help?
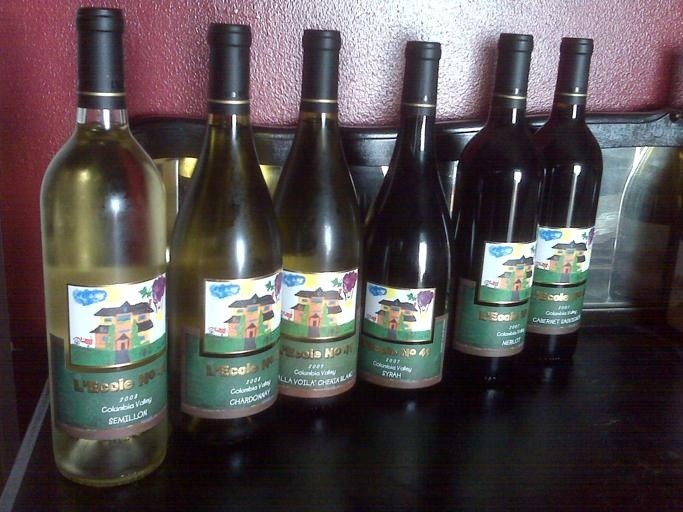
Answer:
[450,33,534,361]
[173,24,287,420]
[527,39,603,335]
[362,40,449,391]
[609,145,677,308]
[51,6,172,480]
[666,150,683,333]
[274,30,364,397]
